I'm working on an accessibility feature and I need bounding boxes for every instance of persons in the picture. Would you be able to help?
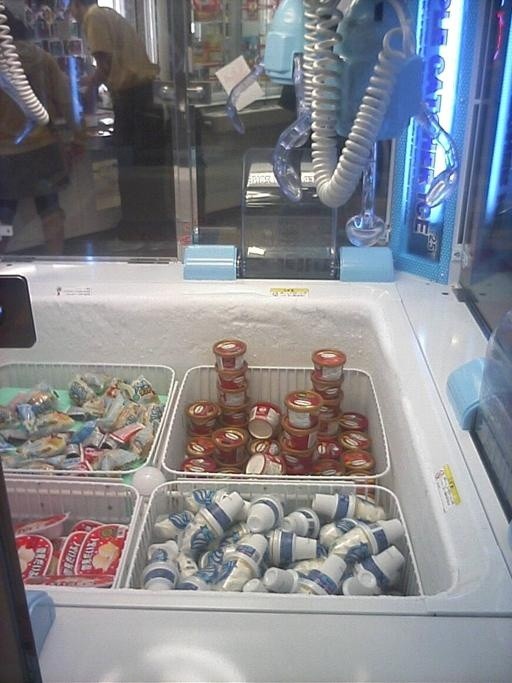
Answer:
[70,0,170,243]
[0,4,76,256]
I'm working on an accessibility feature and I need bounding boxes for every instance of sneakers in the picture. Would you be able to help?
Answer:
[105,237,169,252]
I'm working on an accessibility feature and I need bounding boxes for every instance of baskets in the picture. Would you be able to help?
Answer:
[0,363,176,474]
[6,478,141,590]
[123,478,425,599]
[161,366,391,479]
